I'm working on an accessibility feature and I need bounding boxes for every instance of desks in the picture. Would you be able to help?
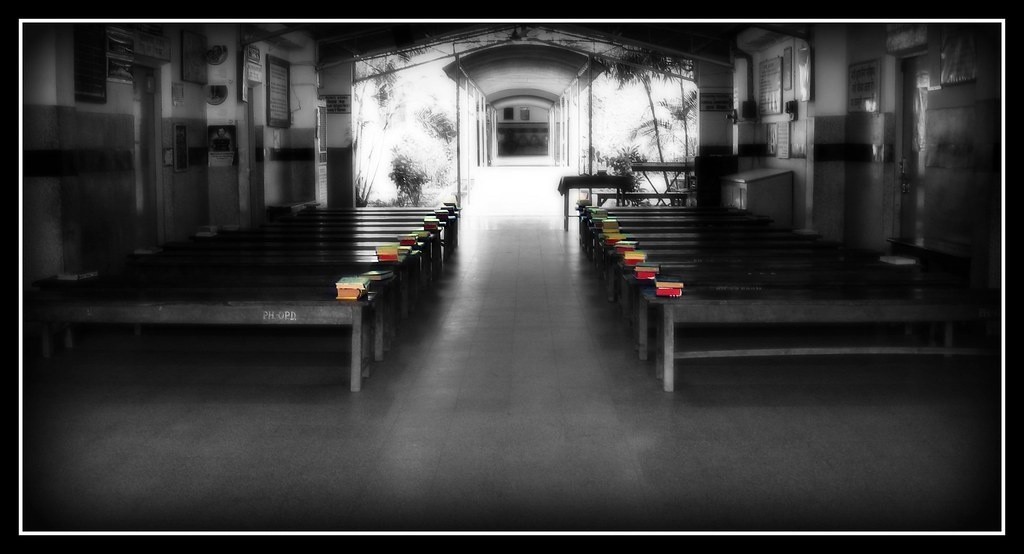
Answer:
[560,176,632,232]
[615,162,695,206]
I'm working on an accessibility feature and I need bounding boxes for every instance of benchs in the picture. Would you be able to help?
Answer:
[597,192,688,207]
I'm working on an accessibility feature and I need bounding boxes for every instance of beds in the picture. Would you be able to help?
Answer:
[39,205,457,392]
[577,206,1001,392]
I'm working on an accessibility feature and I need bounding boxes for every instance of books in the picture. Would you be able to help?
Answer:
[398,246,412,254]
[361,271,393,280]
[603,233,626,245]
[435,203,457,218]
[56,269,98,280]
[576,199,607,222]
[623,250,647,265]
[135,246,162,253]
[602,220,620,235]
[424,219,439,231]
[336,277,370,299]
[615,241,639,252]
[195,226,218,236]
[223,224,240,230]
[632,263,661,279]
[377,245,398,261]
[400,231,430,245]
[597,170,607,175]
[655,277,683,296]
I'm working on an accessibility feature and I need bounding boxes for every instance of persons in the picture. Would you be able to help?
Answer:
[212,128,230,151]
